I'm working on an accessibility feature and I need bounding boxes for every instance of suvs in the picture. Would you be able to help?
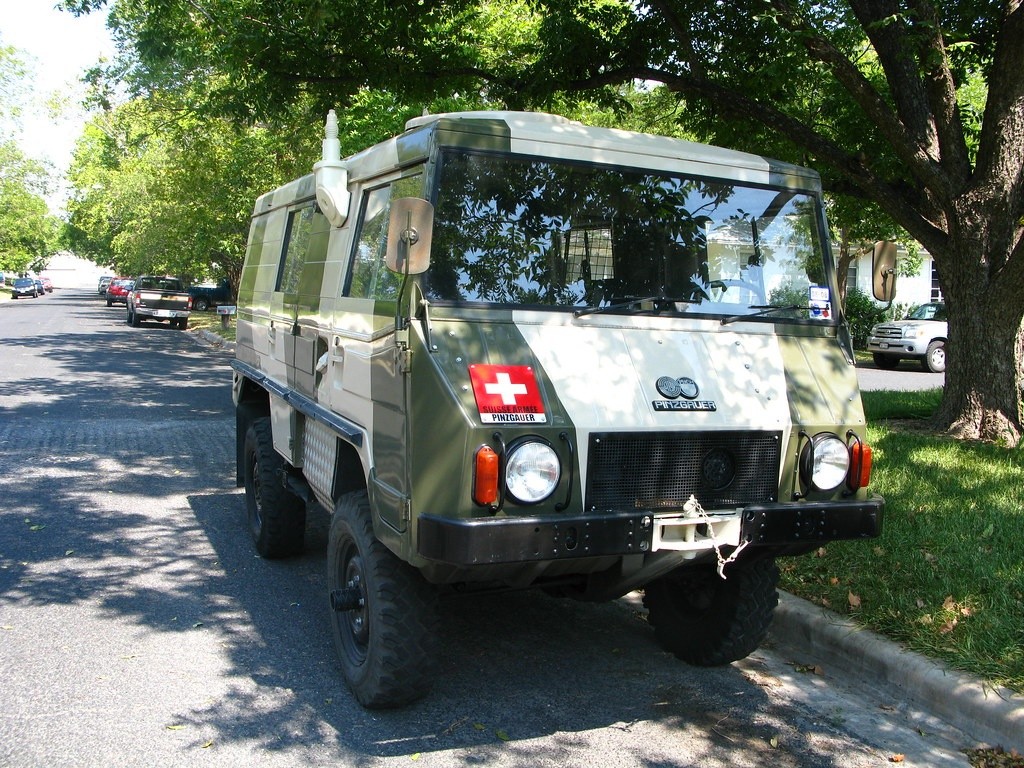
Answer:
[98,277,136,307]
[867,301,948,374]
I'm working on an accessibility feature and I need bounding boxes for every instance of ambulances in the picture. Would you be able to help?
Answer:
[229,110,897,708]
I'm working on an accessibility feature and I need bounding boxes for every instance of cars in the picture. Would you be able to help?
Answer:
[11,277,53,299]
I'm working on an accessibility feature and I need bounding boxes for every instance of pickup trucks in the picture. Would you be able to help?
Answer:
[187,277,239,311]
[126,275,193,331]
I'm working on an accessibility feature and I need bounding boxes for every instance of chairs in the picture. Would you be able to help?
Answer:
[165,283,176,290]
[142,282,152,288]
